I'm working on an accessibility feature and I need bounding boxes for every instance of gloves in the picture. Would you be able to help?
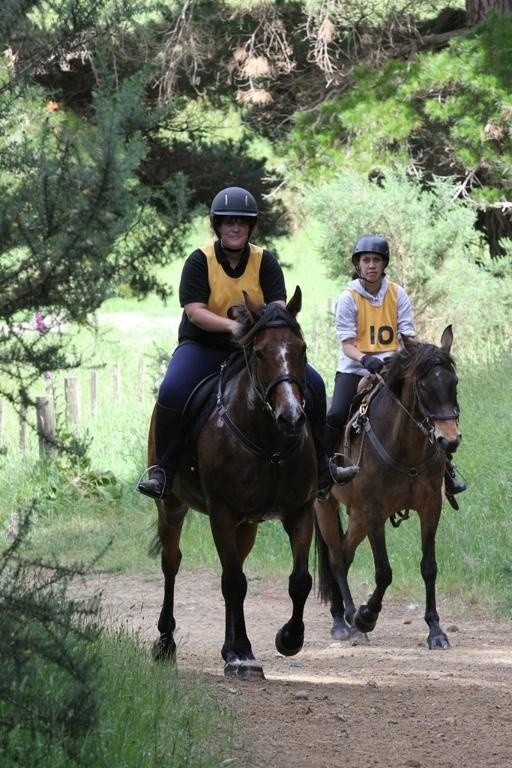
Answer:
[360,355,383,374]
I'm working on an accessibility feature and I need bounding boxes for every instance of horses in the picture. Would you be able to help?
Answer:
[312,324,463,651]
[147,284,320,684]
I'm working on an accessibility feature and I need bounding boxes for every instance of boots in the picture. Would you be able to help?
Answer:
[138,400,183,496]
[446,453,467,494]
[307,406,359,484]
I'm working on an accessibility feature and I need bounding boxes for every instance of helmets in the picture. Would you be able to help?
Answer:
[352,235,389,268]
[209,187,259,220]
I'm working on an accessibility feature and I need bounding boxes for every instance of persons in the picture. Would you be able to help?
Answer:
[138,187,360,499]
[317,236,467,498]
